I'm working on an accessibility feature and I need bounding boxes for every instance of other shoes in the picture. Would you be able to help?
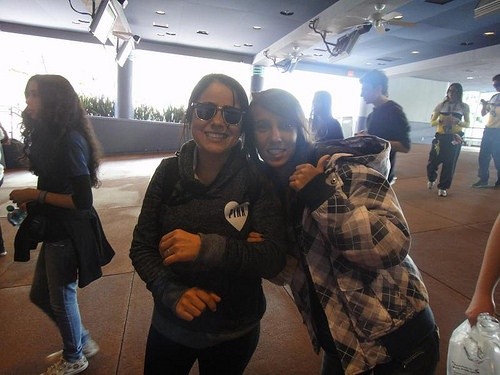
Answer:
[472,180,488,188]
[427,179,437,192]
[438,189,447,197]
[46,339,100,365]
[40,353,88,375]
[494,182,500,189]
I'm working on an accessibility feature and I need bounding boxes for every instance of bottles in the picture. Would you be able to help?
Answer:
[6,205,26,225]
[446,313,500,375]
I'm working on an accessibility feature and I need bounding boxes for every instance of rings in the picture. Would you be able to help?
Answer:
[166,248,171,256]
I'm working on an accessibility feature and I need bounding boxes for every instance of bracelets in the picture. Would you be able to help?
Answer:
[38,190,47,205]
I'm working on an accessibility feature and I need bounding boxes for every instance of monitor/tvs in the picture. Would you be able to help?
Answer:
[286,56,296,72]
[115,41,132,67]
[328,29,361,64]
[88,0,118,45]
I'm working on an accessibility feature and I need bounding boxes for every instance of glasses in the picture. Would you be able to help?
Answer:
[190,102,247,125]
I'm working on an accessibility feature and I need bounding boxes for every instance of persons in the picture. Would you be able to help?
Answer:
[0,75,116,375]
[426,83,469,197]
[359,70,411,186]
[472,74,500,189]
[309,91,344,146]
[128,73,289,375]
[464,209,500,375]
[240,88,441,375]
